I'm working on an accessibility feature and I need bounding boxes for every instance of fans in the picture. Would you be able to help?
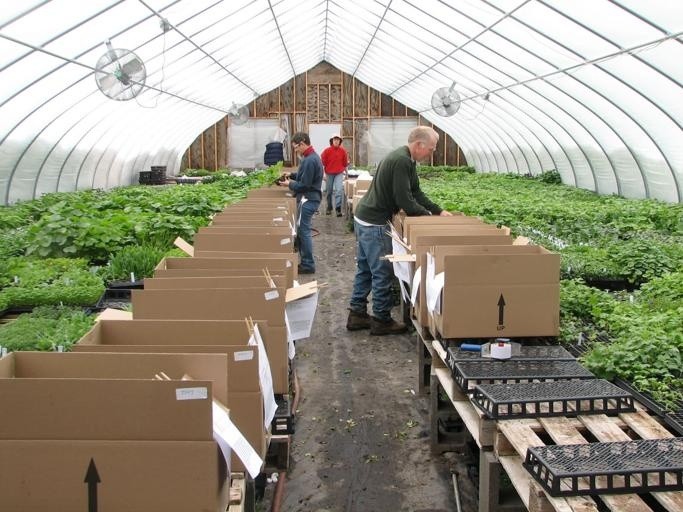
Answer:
[432,82,489,121]
[93,18,170,110]
[228,103,250,126]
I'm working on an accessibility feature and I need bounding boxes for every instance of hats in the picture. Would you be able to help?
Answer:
[329,134,343,145]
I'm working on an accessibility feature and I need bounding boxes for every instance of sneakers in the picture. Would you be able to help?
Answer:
[336,207,342,217]
[346,308,374,330]
[326,206,333,215]
[298,263,315,274]
[369,316,408,336]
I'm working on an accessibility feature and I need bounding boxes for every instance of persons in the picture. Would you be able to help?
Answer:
[321,136,350,216]
[346,126,453,336]
[279,132,323,274]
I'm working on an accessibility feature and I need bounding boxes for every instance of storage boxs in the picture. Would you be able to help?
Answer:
[342,170,561,339]
